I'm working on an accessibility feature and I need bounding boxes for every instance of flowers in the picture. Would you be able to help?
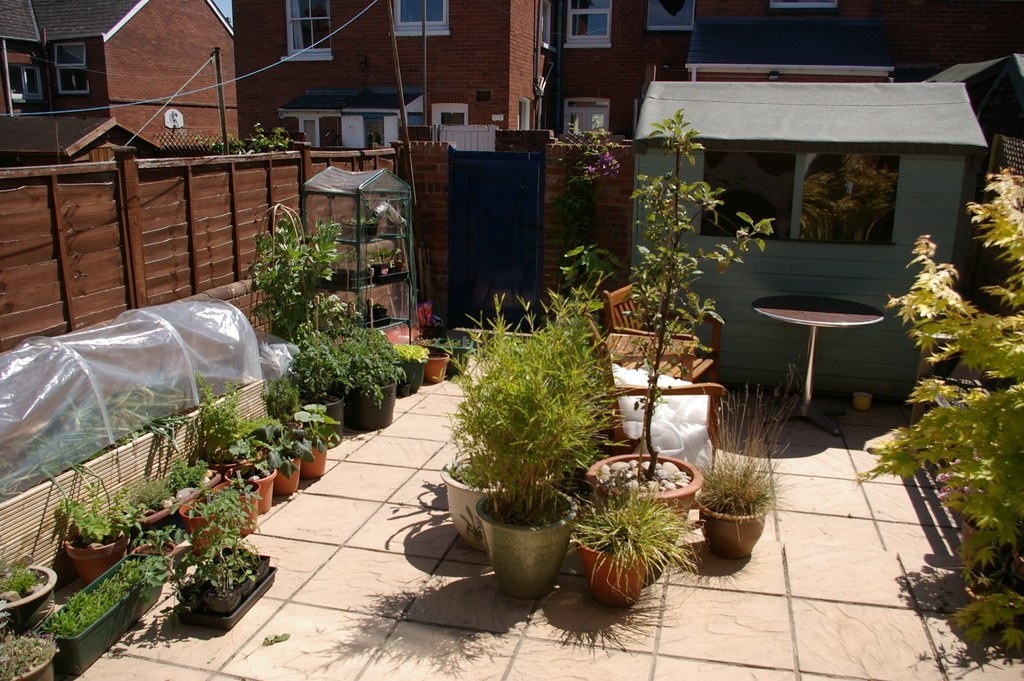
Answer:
[935,458,994,515]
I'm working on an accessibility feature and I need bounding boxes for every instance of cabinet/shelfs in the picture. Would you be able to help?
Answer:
[303,166,413,344]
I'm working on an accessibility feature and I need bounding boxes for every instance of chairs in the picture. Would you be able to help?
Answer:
[602,283,724,383]
[588,318,726,479]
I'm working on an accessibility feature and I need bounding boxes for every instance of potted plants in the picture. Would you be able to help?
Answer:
[212,480,262,536]
[0,550,58,640]
[294,404,342,477]
[225,436,281,515]
[178,499,225,556]
[58,481,147,587]
[261,375,302,431]
[35,554,168,677]
[0,630,61,681]
[455,325,636,600]
[440,283,605,552]
[254,202,468,430]
[194,371,252,478]
[120,456,222,547]
[962,560,1004,606]
[694,363,800,559]
[561,482,701,607]
[251,422,317,494]
[160,530,270,614]
[585,107,776,588]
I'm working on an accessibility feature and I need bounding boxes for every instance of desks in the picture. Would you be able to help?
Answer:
[749,296,886,437]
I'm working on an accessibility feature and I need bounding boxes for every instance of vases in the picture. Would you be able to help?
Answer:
[1013,549,1024,578]
[961,516,1003,560]
